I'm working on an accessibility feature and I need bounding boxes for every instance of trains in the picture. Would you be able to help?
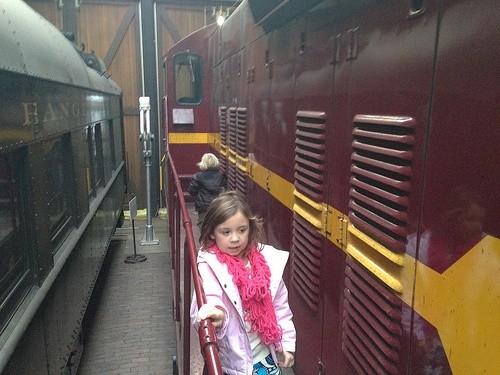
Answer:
[1,0,499,375]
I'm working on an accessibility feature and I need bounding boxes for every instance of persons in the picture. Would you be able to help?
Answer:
[188,188,299,375]
[188,154,228,229]
